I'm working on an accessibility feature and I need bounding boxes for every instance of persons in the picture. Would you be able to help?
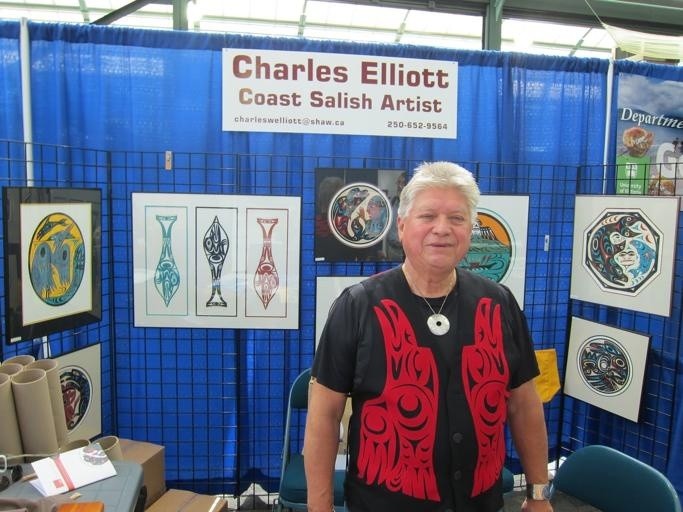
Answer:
[304,162,556,512]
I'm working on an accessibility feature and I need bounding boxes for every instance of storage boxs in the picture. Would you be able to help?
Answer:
[117,439,228,512]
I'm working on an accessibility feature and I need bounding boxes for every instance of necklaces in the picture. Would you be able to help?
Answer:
[404,270,454,337]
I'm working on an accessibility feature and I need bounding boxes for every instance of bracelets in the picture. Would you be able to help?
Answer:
[527,484,550,501]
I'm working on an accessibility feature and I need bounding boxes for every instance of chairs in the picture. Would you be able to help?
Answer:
[542,444,681,511]
[276,369,352,511]
